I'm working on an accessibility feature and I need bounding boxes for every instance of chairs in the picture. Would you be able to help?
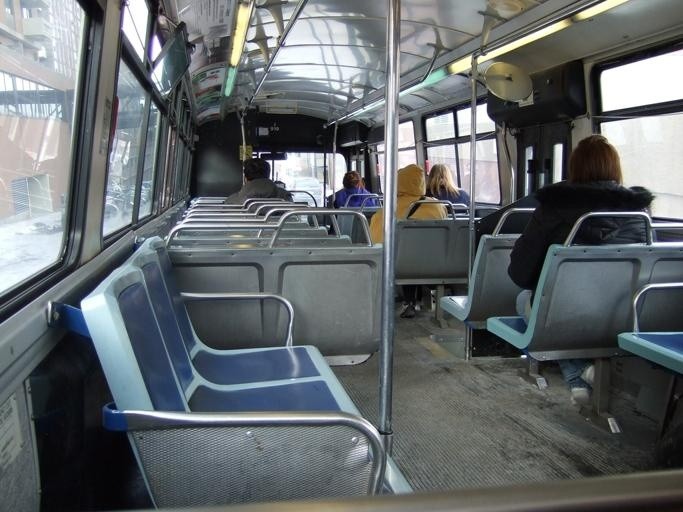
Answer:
[329,194,482,331]
[436,208,549,386]
[616,284,682,373]
[82,235,414,511]
[488,212,682,437]
[163,195,384,369]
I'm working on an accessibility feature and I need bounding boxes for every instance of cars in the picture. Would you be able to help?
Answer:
[290,177,322,204]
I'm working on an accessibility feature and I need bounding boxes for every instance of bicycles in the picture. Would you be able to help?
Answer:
[104,181,152,227]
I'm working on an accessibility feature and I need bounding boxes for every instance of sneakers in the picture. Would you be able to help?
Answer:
[580,365,595,388]
[570,386,591,406]
[400,303,421,318]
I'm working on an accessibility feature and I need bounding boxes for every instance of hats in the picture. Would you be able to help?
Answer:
[243,158,270,179]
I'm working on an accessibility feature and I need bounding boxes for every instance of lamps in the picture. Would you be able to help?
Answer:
[356,0,630,114]
[221,0,256,97]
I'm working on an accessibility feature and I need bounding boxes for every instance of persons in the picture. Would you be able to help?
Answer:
[425,163,470,213]
[328,171,380,236]
[222,157,293,216]
[368,163,448,319]
[504,134,656,406]
